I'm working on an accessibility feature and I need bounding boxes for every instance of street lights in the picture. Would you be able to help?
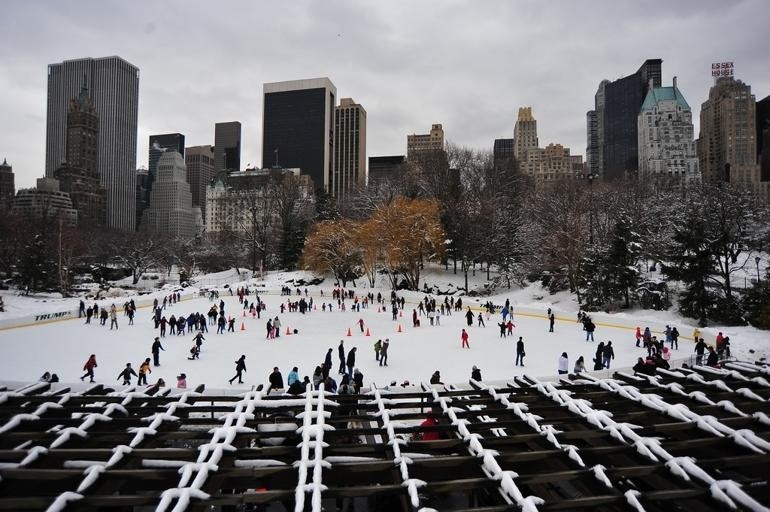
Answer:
[249,206,258,274]
[586,173,599,245]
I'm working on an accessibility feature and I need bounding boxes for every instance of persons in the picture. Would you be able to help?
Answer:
[421,410,441,441]
[430,371,440,384]
[269,339,363,398]
[374,338,389,366]
[151,292,235,360]
[0,295,6,312]
[152,337,166,366]
[546,307,731,376]
[279,286,516,349]
[409,427,423,442]
[80,354,97,383]
[47,374,59,383]
[472,365,482,381]
[228,355,247,385]
[38,371,50,382]
[200,288,219,301]
[177,373,187,388]
[157,377,166,387]
[227,285,282,339]
[390,380,409,388]
[516,336,525,366]
[77,298,136,330]
[117,357,151,386]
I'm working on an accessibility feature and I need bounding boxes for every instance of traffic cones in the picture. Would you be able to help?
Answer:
[241,322,246,330]
[365,327,370,336]
[228,316,231,322]
[286,327,292,335]
[346,327,352,336]
[399,310,403,317]
[313,304,317,310]
[242,310,246,317]
[377,307,381,312]
[397,324,403,332]
